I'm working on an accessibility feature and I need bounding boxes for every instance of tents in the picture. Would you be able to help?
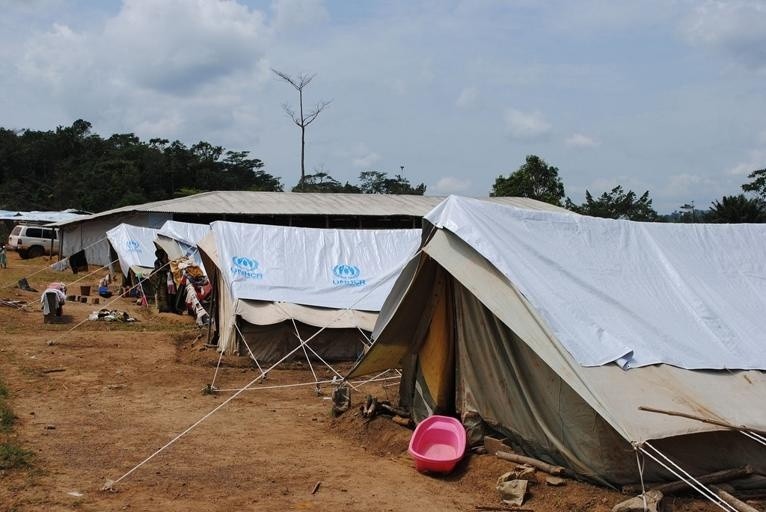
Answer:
[42,193,765,492]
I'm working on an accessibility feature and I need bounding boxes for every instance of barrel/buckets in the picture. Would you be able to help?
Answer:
[79,284,92,296]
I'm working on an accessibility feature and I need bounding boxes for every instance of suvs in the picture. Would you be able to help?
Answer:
[4,225,59,258]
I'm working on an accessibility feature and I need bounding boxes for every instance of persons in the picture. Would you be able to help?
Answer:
[0,246,7,268]
